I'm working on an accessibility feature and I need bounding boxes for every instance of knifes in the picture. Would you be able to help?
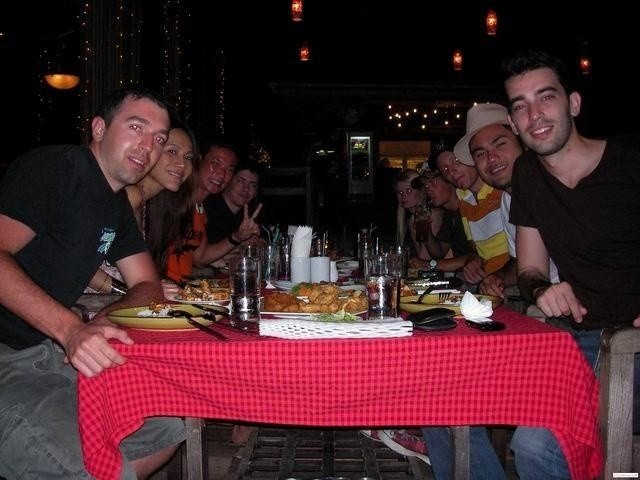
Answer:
[167,301,265,341]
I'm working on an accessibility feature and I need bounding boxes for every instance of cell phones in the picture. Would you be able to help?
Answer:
[465,319,506,331]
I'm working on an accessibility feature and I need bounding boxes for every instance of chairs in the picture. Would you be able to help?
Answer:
[526,303,640,480]
[69,303,182,480]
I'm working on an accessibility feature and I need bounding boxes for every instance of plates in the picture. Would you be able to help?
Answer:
[167,276,229,304]
[104,303,227,333]
[401,275,506,317]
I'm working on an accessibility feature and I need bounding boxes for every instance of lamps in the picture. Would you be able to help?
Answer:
[486,10,498,36]
[452,49,463,71]
[300,46,310,62]
[45,71,80,91]
[292,0,302,22]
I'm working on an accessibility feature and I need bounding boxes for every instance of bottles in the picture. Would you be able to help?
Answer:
[229,226,409,328]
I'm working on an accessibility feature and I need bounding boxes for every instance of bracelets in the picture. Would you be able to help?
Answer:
[97,273,109,292]
[228,232,242,247]
[110,277,128,296]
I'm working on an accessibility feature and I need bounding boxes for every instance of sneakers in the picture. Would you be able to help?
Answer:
[376,428,431,465]
[360,428,381,443]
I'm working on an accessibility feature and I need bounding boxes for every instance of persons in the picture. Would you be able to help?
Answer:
[359,102,559,466]
[204,161,270,276]
[427,136,510,295]
[162,136,264,288]
[1,87,187,480]
[83,121,200,321]
[419,50,640,480]
[392,167,455,261]
[408,168,479,273]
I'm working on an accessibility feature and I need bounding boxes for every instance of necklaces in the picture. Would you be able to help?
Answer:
[136,181,148,241]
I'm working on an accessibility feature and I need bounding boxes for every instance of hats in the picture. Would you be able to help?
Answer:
[427,139,453,170]
[453,102,510,167]
[412,160,433,190]
